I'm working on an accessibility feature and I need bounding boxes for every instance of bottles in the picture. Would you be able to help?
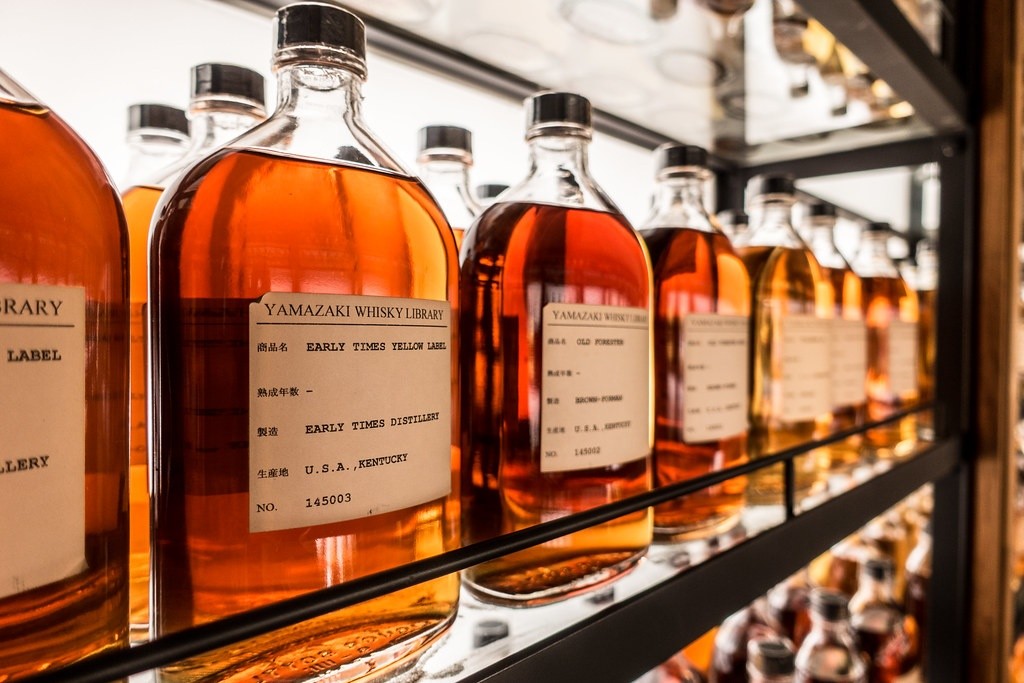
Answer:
[0,2,938,683]
[564,0,944,114]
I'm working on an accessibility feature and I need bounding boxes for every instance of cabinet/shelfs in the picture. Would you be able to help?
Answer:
[1,0,1024,683]
